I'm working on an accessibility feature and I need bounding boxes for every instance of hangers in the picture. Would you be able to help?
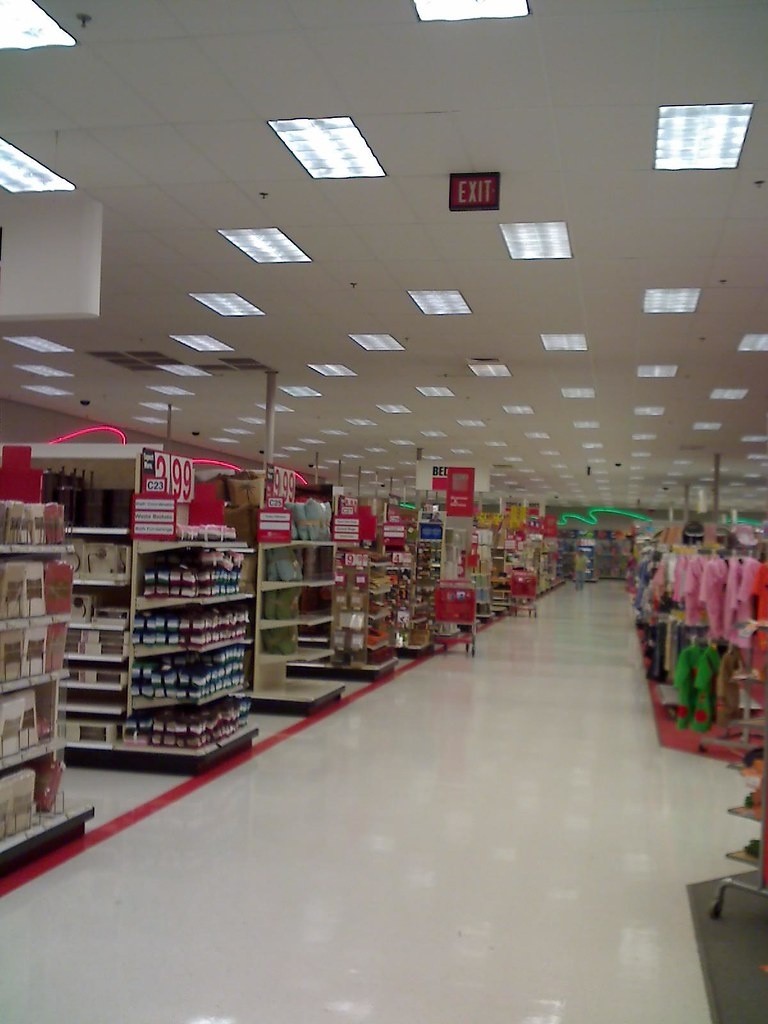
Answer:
[683,636,701,650]
[722,637,737,659]
[671,543,757,560]
[704,636,720,657]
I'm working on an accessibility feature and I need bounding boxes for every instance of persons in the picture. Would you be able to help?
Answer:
[574,550,588,591]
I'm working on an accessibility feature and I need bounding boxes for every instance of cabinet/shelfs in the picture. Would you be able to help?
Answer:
[249,536,347,718]
[331,529,576,674]
[0,540,97,881]
[61,520,260,765]
[628,547,766,708]
[726,611,766,868]
